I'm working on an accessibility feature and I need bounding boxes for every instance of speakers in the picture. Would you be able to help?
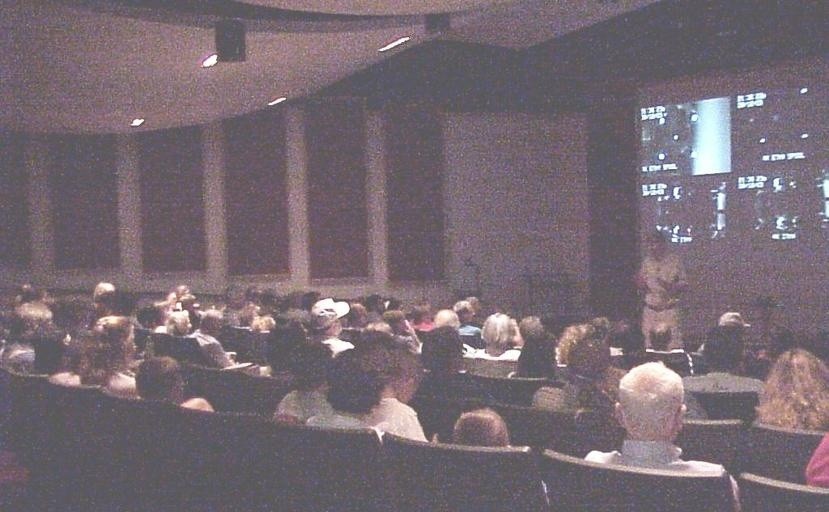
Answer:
[215,19,246,62]
[423,14,450,32]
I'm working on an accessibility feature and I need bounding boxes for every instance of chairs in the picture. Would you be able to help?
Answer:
[0,287,828,511]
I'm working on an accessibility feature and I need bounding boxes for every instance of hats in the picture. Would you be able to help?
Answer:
[718,311,751,327]
[310,299,350,329]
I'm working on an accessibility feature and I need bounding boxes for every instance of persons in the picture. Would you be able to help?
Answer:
[447,294,829,510]
[4,278,522,446]
[633,232,691,350]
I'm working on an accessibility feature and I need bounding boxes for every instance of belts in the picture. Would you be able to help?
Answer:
[647,303,675,311]
